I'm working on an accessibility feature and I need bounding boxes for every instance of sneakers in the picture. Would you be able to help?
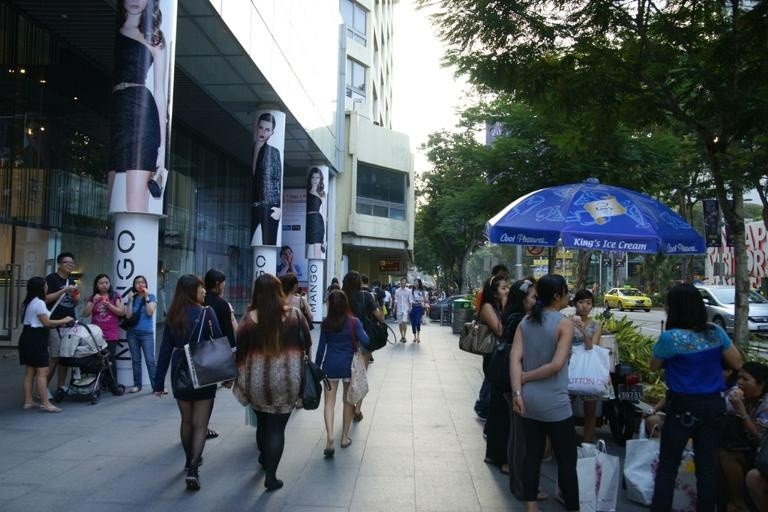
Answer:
[58,386,69,392]
[474,400,487,422]
[205,429,218,437]
[354,412,362,421]
[185,472,201,489]
[129,386,139,392]
[36,389,53,401]
[185,456,203,471]
[153,387,168,394]
[265,478,285,489]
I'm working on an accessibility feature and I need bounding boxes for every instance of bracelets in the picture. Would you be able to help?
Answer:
[742,414,751,421]
[511,390,522,398]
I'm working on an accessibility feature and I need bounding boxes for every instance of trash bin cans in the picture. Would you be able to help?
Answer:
[451,298,472,336]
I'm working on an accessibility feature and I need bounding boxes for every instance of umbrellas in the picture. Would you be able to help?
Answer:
[482,176,706,317]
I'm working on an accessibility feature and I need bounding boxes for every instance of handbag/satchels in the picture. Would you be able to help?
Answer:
[362,291,388,352]
[347,316,368,408]
[673,447,695,512]
[485,313,524,393]
[119,293,145,330]
[595,439,619,511]
[755,429,768,475]
[624,420,661,505]
[184,306,238,389]
[383,298,388,314]
[149,166,163,202]
[299,297,315,330]
[576,443,597,511]
[49,277,68,319]
[460,303,502,354]
[717,414,751,452]
[293,308,331,410]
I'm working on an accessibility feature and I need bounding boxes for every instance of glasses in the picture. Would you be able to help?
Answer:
[59,261,73,264]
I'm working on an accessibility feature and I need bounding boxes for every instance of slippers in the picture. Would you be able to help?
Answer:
[341,436,352,448]
[324,448,335,456]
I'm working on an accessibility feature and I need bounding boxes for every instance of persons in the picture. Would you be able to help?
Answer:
[718,360,767,511]
[649,281,742,512]
[245,112,281,245]
[474,263,510,311]
[384,287,392,308]
[393,278,412,343]
[305,167,329,257]
[410,278,430,344]
[36,252,81,399]
[327,278,340,290]
[280,274,313,322]
[720,360,739,418]
[500,279,548,500]
[83,272,125,380]
[566,290,601,442]
[478,277,509,473]
[277,247,303,277]
[126,275,157,393]
[314,292,370,456]
[509,274,579,512]
[234,274,312,489]
[372,281,386,309]
[746,466,768,511]
[342,270,385,419]
[390,284,397,323]
[423,286,429,325]
[430,289,435,303]
[17,276,74,413]
[201,267,233,345]
[361,276,373,297]
[107,0,169,214]
[154,274,233,490]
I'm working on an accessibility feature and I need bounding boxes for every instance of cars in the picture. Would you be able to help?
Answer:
[429,295,464,323]
[695,284,768,335]
[603,288,652,312]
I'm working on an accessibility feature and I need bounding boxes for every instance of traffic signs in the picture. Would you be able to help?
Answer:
[525,245,545,256]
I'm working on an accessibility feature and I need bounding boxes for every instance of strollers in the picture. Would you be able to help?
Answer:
[55,318,124,404]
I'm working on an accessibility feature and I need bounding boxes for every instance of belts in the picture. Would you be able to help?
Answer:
[112,83,147,96]
[306,211,320,215]
[251,201,278,208]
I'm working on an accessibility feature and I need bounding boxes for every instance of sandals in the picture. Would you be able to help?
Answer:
[23,402,39,410]
[40,403,63,412]
